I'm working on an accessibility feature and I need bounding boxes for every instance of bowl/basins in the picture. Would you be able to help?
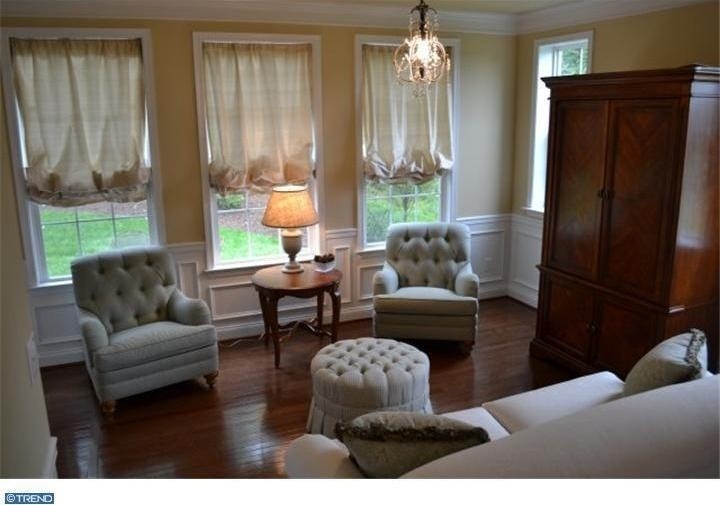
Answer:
[312,259,337,273]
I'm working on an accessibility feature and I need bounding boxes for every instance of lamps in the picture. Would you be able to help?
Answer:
[391,0,450,100]
[261,185,319,274]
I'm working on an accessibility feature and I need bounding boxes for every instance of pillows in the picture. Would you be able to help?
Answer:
[623,328,707,398]
[334,413,491,478]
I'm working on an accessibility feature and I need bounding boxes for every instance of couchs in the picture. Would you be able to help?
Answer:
[285,369,720,479]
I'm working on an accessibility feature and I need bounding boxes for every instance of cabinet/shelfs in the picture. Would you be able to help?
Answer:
[529,65,720,382]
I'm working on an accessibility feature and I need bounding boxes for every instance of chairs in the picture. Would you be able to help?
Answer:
[71,244,219,421]
[372,222,481,359]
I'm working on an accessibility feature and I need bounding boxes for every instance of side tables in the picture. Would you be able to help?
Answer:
[251,263,344,369]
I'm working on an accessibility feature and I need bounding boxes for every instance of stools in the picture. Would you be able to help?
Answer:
[306,337,434,441]
